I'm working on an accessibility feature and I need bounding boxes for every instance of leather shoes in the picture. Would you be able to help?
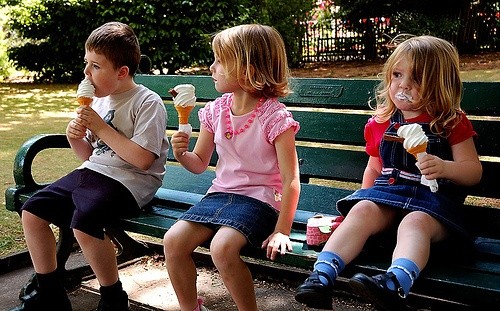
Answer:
[348,271,407,311]
[295,271,333,310]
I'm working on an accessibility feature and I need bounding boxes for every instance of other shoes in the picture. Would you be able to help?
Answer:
[7,290,73,311]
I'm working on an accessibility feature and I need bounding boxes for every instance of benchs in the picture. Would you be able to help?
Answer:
[6,73,500,311]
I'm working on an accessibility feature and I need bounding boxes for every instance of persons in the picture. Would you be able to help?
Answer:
[295,36,483,310]
[164,24,300,311]
[5,22,169,311]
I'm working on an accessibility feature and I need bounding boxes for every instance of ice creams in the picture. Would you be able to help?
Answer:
[396,123,429,160]
[167,84,196,124]
[76,79,95,106]
